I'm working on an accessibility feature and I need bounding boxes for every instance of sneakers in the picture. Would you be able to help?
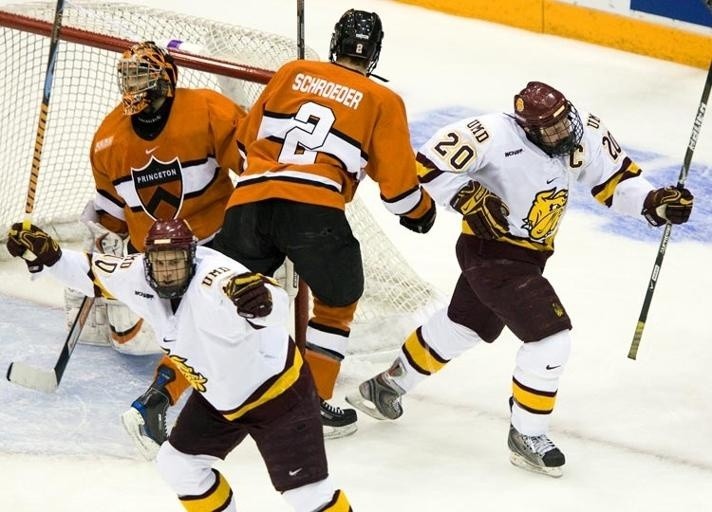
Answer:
[509,426,565,467]
[320,396,357,428]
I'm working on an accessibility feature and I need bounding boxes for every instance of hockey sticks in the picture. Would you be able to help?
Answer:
[7,296,94,393]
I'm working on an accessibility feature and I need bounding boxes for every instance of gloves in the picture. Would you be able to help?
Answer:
[641,186,693,227]
[400,185,436,233]
[222,272,272,320]
[450,180,509,240]
[6,222,61,267]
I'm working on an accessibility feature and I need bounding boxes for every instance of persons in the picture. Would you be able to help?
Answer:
[345,80,694,478]
[7,219,353,512]
[65,41,248,356]
[120,8,437,463]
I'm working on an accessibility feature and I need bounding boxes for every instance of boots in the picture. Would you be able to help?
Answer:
[359,357,406,420]
[130,365,176,446]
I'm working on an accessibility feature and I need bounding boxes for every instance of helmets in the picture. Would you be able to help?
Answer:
[143,219,197,299]
[329,8,384,60]
[117,40,178,115]
[514,81,575,155]
